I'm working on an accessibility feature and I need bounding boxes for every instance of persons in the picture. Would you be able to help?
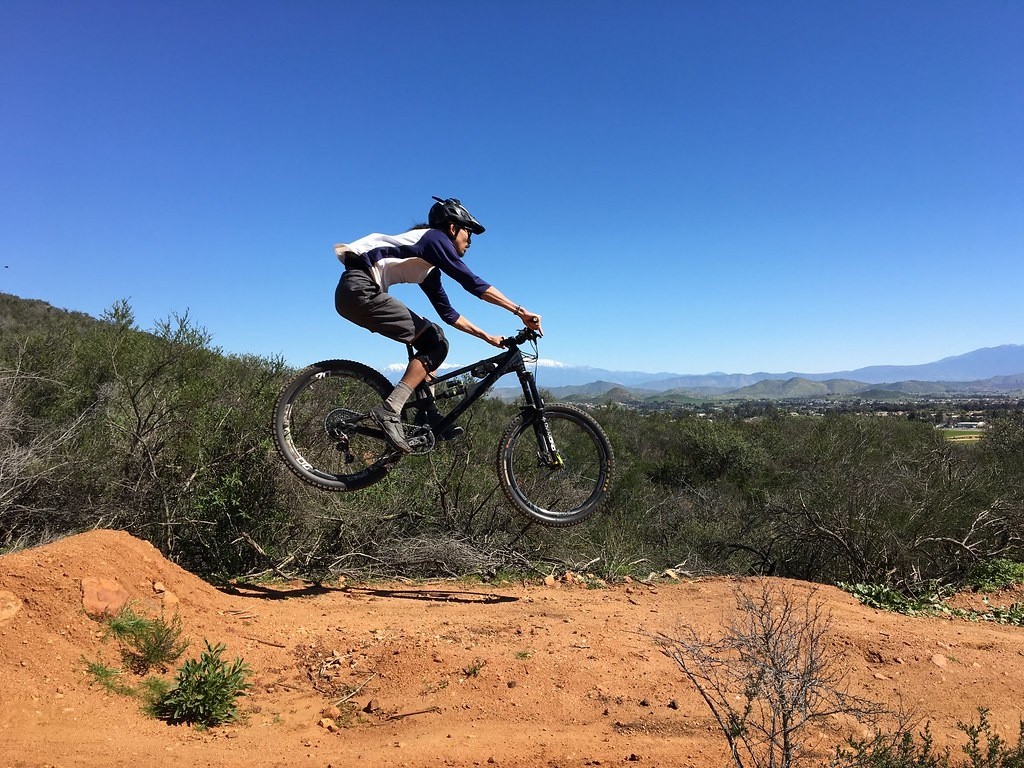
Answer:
[334,195,545,454]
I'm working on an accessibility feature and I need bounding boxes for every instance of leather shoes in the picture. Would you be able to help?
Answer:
[419,410,464,442]
[367,400,413,452]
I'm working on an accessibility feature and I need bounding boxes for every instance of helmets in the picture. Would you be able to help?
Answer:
[429,195,484,235]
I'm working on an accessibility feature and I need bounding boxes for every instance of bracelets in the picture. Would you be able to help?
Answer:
[514,305,521,316]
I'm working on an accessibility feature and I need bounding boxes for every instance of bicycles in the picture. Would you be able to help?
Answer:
[272,313,617,526]
